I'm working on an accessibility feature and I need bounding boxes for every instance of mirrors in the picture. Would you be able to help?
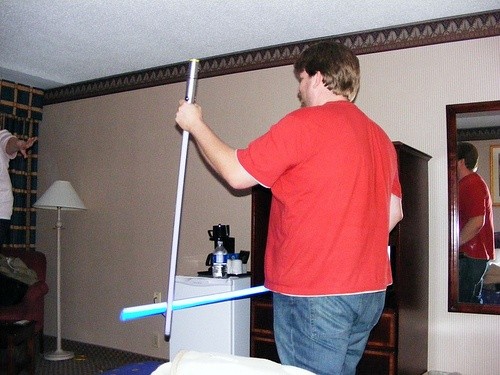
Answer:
[443,99,500,318]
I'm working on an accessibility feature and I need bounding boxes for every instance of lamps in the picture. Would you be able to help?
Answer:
[32,178,90,362]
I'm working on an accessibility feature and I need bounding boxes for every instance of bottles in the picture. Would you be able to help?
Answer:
[212,241,247,278]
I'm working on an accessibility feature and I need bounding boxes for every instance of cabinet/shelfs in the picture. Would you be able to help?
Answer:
[245,140,434,375]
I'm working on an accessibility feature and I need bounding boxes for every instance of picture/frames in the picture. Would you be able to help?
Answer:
[489,144,500,206]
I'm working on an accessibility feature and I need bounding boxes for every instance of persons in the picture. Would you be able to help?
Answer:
[176,42,403,375]
[456,142,494,306]
[0,129,37,243]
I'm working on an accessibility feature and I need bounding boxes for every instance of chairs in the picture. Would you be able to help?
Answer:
[0,245,50,375]
